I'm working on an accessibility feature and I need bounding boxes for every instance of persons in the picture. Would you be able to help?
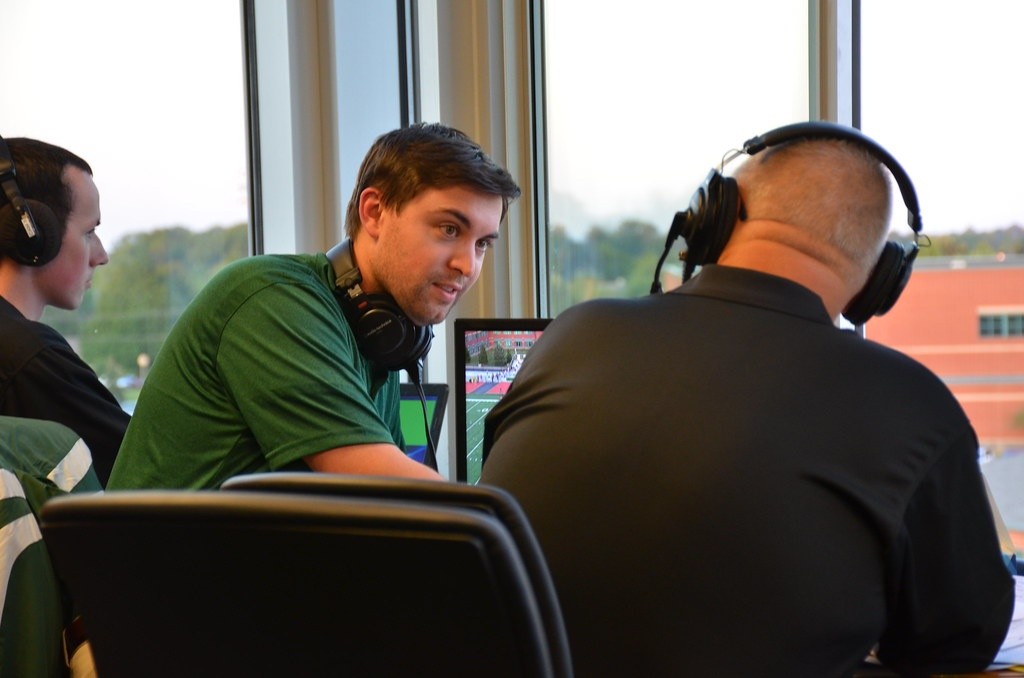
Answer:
[106,123,520,494]
[0,136,131,491]
[478,122,1015,678]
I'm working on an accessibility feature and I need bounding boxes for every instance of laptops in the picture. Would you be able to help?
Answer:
[399,382,448,471]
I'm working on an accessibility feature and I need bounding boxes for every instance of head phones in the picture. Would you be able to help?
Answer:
[677,119,931,328]
[324,237,435,385]
[0,135,62,266]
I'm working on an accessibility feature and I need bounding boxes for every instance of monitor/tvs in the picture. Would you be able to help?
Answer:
[454,320,565,487]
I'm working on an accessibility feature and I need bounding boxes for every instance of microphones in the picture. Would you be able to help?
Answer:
[650,211,685,298]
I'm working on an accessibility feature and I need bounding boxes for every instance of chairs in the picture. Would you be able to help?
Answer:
[0,418,573,678]
